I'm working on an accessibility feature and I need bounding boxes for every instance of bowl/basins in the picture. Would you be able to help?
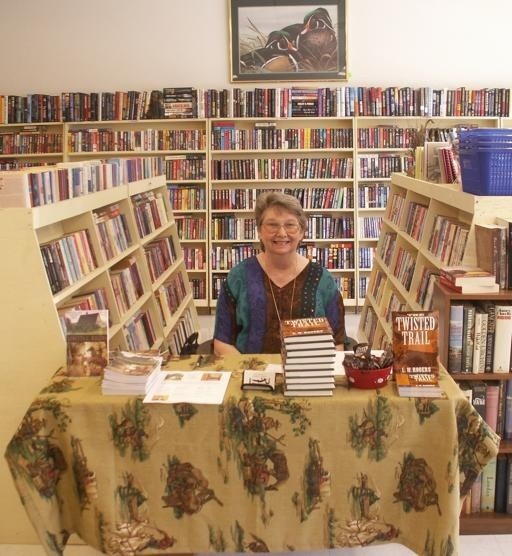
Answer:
[342,362,393,389]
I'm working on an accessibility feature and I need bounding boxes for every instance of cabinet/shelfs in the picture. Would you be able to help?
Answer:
[355,117,501,306]
[1,157,199,361]
[0,123,65,170]
[357,171,512,535]
[208,118,356,308]
[65,118,209,309]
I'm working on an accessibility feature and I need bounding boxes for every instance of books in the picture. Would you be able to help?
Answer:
[281,317,337,397]
[64,311,108,377]
[211,88,512,300]
[0,87,206,357]
[102,350,162,396]
[241,370,276,391]
[359,268,512,517]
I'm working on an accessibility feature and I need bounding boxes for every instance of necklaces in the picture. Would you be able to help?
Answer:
[263,257,294,327]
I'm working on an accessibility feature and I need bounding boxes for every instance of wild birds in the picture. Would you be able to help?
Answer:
[240,29,304,72]
[281,7,337,71]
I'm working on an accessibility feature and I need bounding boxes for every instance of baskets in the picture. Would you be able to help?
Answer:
[456,123,512,196]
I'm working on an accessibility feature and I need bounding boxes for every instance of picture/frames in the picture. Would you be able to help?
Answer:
[227,0,348,83]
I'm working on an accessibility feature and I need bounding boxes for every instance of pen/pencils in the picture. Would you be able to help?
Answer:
[197,356,203,367]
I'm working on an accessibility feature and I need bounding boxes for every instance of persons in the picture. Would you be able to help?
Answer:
[213,190,345,359]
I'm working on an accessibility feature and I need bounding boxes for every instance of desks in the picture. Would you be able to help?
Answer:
[1,351,502,556]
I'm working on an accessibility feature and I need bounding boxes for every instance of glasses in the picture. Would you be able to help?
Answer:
[261,218,301,233]
